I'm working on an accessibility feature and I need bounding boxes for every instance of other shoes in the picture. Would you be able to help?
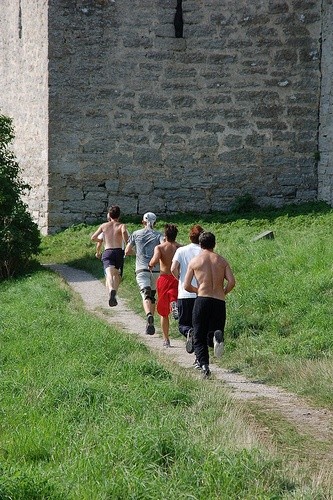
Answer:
[185,328,195,354]
[145,315,155,335]
[172,301,179,319]
[200,369,211,377]
[109,290,118,307]
[162,340,170,346]
[192,361,200,369]
[214,330,223,357]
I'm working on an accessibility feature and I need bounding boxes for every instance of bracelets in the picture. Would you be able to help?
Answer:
[147,264,154,268]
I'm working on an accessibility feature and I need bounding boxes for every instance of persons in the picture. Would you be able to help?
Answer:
[91,205,129,306]
[125,213,166,335]
[171,226,205,370]
[96,212,130,289]
[148,223,183,347]
[184,232,235,381]
